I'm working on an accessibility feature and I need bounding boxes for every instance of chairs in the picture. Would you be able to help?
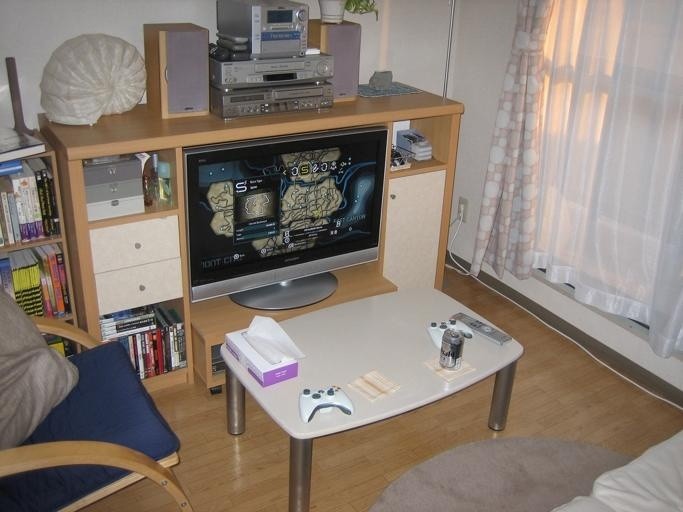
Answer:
[0,311,194,512]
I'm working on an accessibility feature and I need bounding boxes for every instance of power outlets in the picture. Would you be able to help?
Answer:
[456,195,469,223]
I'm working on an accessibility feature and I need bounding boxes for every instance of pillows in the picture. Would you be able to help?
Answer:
[0,284,80,451]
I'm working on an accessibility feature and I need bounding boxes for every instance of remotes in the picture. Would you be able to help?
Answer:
[452,313,512,345]
[208,32,249,61]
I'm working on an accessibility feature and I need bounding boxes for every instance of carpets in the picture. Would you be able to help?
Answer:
[371,436,634,512]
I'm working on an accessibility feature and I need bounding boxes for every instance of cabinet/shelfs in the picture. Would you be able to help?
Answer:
[380,81,465,291]
[175,263,399,390]
[38,107,196,392]
[0,129,83,355]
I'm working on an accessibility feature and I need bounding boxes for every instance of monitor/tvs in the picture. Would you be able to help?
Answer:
[182,126,389,310]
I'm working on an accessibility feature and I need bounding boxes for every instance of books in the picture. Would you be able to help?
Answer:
[99,303,188,382]
[43,334,77,359]
[0,243,70,318]
[1,158,62,246]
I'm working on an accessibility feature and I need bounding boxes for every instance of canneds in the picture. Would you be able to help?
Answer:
[439,328,464,370]
